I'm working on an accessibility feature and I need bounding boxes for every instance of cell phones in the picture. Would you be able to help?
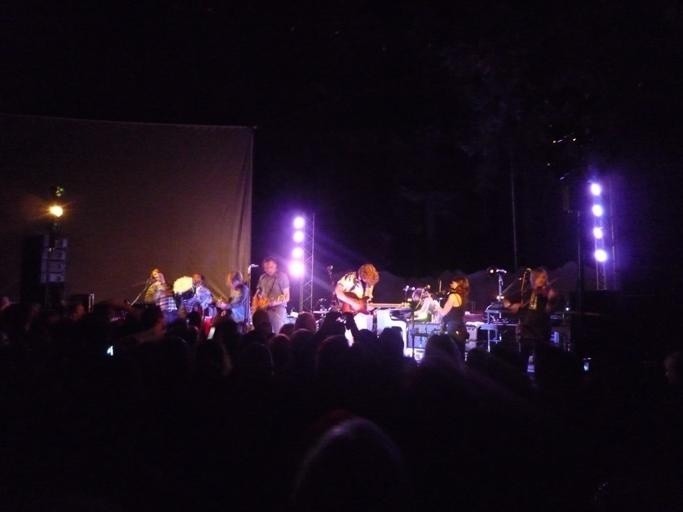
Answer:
[207,326,216,340]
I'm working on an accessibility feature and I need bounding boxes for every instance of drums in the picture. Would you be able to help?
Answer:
[172,277,195,300]
[179,297,199,315]
[156,294,179,312]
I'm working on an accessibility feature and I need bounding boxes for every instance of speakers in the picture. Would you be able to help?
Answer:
[71,293,95,313]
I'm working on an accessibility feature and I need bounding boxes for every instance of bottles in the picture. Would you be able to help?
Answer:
[527,356,535,377]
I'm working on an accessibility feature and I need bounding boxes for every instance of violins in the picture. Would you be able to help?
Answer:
[544,280,560,300]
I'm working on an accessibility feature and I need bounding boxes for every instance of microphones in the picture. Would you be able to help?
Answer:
[524,267,531,274]
[486,265,507,280]
[403,287,415,291]
[326,264,333,273]
[251,262,259,268]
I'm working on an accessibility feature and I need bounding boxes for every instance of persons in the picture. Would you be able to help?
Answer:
[1,295,683,512]
[411,288,441,324]
[436,275,469,362]
[145,269,216,325]
[219,272,250,333]
[254,256,290,335]
[512,266,556,374]
[334,264,379,347]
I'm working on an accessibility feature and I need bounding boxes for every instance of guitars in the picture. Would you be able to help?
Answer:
[252,294,284,315]
[342,292,408,316]
